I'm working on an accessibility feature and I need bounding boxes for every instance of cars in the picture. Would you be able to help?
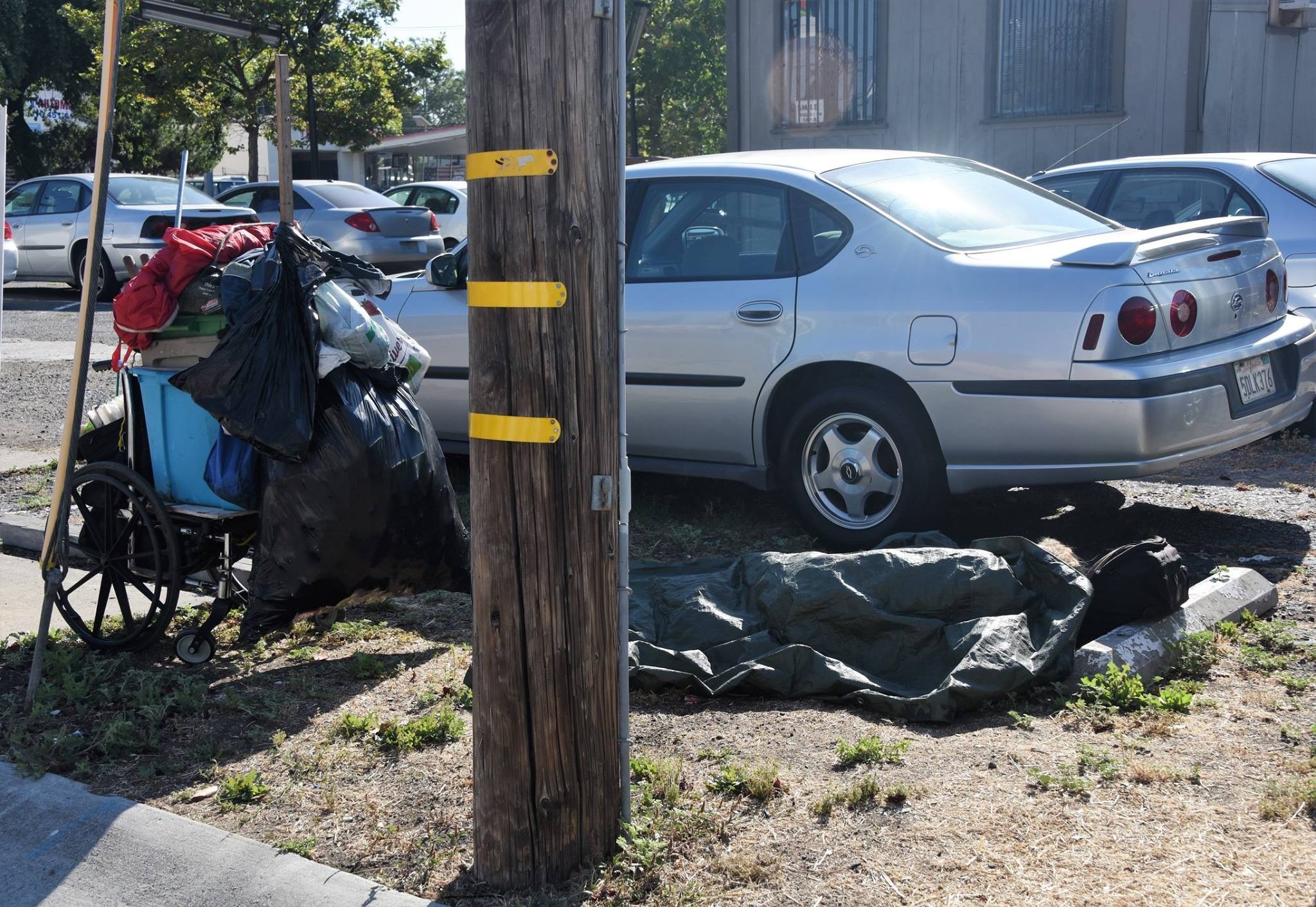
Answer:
[377,180,468,250]
[205,177,447,282]
[1014,152,1315,331]
[357,146,1315,550]
[5,173,264,303]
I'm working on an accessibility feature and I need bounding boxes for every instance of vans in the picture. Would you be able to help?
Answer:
[185,174,250,201]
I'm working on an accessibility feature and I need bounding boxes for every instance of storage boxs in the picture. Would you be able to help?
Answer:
[125,314,254,514]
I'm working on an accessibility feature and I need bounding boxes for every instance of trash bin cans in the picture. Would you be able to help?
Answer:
[128,366,248,512]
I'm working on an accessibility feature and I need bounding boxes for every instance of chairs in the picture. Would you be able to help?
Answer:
[45,190,78,214]
[1196,208,1221,220]
[683,230,742,279]
[1139,208,1177,230]
[424,197,444,216]
[814,230,842,258]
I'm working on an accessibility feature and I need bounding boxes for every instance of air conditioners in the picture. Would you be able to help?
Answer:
[393,155,409,167]
